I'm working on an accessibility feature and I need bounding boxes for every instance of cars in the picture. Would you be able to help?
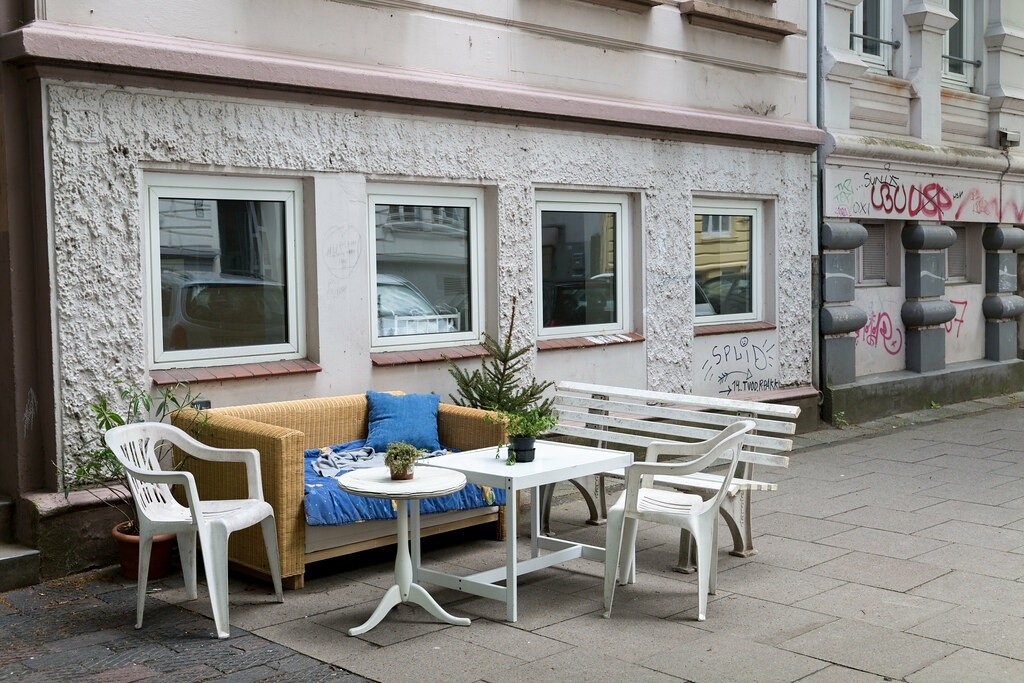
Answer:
[377,274,457,337]
[160,268,287,349]
[545,273,751,327]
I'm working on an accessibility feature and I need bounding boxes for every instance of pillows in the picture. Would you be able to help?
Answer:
[364,390,440,450]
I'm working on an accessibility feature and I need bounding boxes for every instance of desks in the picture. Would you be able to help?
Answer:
[338,466,473,637]
[409,439,637,624]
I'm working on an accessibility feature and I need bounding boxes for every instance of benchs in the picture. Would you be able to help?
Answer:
[170,391,520,592]
[538,381,802,575]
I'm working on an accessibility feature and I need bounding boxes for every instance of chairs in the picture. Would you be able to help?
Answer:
[601,420,755,623]
[104,422,285,640]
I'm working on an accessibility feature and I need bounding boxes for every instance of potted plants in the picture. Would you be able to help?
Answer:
[385,440,428,481]
[50,379,212,580]
[483,403,560,467]
